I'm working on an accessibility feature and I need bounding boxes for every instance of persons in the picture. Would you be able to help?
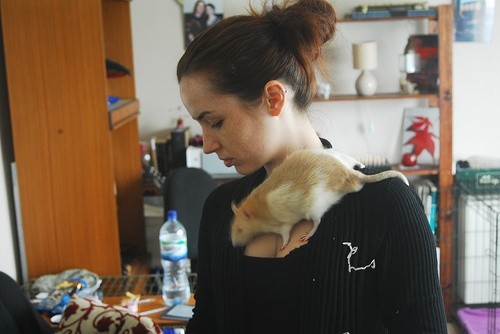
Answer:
[186,0,220,42]
[176,0,446,334]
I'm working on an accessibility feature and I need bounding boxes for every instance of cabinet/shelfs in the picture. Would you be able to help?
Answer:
[1,0,152,285]
[304,6,455,321]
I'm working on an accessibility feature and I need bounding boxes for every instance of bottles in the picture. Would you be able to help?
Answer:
[159,210,190,307]
